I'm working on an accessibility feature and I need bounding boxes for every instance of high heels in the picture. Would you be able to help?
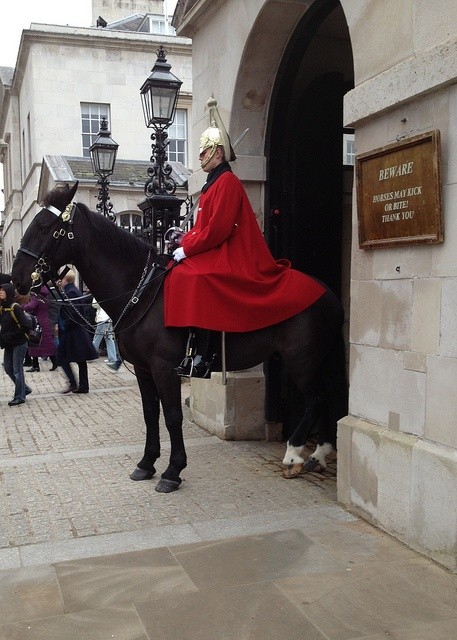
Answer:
[26,362,40,372]
[49,358,61,371]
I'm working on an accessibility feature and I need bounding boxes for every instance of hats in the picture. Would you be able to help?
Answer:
[198,89,237,168]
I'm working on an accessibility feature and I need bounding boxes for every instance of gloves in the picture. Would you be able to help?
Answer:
[172,246,187,263]
[170,230,186,244]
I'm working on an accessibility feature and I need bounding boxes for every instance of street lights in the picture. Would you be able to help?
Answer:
[137,46,184,254]
[89,115,119,222]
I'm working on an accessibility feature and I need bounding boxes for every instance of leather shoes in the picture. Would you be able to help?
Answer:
[173,355,211,380]
[72,389,89,393]
[64,387,77,392]
[14,391,31,396]
[8,398,25,406]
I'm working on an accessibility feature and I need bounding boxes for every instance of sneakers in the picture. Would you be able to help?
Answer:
[106,363,118,371]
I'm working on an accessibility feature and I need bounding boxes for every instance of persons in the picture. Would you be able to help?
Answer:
[22,280,58,372]
[54,269,100,393]
[47,280,62,332]
[90,294,116,365]
[106,356,125,370]
[163,127,327,380]
[12,285,30,305]
[0,283,32,407]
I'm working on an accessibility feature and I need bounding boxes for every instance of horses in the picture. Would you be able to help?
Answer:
[11,179,345,494]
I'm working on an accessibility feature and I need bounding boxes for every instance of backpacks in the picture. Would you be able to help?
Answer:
[24,313,42,347]
